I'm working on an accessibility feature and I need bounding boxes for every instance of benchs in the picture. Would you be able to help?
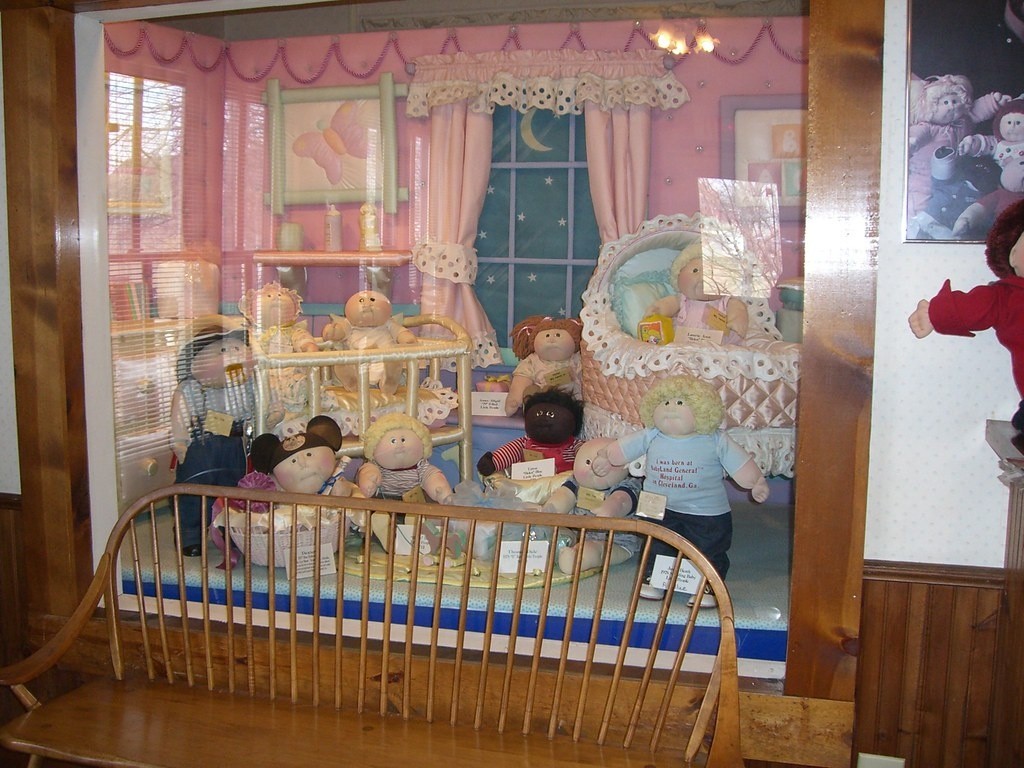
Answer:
[0,484,744,768]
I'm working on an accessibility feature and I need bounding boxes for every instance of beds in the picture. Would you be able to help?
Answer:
[258,314,473,487]
[579,211,803,482]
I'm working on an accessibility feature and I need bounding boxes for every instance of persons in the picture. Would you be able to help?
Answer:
[251,415,365,531]
[907,72,1024,241]
[239,283,319,428]
[546,438,643,573]
[909,199,1024,430]
[504,316,584,417]
[477,386,588,479]
[644,243,747,348]
[356,412,452,552]
[322,291,416,395]
[173,326,252,557]
[595,375,771,607]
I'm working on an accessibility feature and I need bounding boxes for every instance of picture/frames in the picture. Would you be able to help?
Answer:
[260,70,408,213]
[902,0,1024,243]
[718,94,808,221]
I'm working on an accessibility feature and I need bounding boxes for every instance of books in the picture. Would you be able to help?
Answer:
[110,281,150,320]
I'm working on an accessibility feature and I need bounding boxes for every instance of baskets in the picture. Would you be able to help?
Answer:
[230,515,349,567]
